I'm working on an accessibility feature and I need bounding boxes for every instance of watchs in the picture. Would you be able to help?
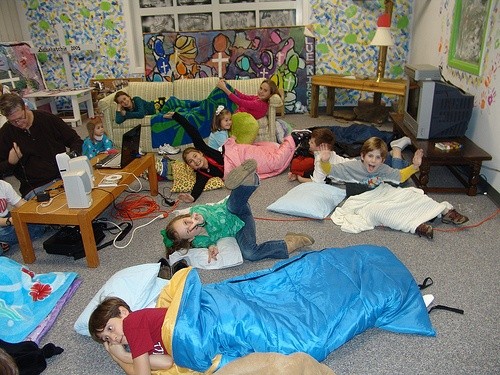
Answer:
[6,217,11,226]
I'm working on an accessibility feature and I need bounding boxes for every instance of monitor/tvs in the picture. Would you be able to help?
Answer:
[403,76,474,140]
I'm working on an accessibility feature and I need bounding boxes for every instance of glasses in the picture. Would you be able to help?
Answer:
[6,107,26,124]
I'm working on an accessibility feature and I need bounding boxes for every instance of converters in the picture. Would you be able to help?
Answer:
[165,198,176,205]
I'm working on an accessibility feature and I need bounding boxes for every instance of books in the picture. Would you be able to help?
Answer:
[435,142,463,150]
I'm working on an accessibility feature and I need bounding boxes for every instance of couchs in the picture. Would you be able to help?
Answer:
[98,77,283,152]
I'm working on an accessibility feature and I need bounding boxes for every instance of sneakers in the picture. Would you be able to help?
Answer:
[157,143,181,155]
[390,135,412,151]
[416,223,434,239]
[442,209,469,225]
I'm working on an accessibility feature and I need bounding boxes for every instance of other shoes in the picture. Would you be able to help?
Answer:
[291,128,312,140]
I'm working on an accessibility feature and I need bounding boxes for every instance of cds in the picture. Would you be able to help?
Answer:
[105,175,122,180]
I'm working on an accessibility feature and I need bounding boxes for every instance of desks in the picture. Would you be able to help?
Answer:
[11,152,158,268]
[311,74,407,119]
[23,87,95,126]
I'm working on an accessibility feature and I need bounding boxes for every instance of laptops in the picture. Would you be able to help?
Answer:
[95,124,141,169]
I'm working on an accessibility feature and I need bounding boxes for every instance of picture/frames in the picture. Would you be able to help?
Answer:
[448,0,493,76]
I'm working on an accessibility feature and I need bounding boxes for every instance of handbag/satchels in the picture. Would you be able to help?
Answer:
[43,220,132,261]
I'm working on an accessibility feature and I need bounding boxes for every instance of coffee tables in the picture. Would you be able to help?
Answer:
[390,112,492,197]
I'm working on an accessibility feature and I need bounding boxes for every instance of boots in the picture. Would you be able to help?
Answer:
[284,231,315,254]
[225,159,258,190]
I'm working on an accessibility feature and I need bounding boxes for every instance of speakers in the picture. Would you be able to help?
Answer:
[62,170,93,208]
[69,156,94,189]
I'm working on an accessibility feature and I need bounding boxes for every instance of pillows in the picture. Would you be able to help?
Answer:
[169,194,243,271]
[74,263,161,337]
[266,181,347,219]
[229,112,258,144]
[170,162,225,192]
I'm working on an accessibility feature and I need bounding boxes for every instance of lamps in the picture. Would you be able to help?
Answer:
[371,27,393,83]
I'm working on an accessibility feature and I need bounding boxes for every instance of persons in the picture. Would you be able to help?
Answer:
[162,110,312,203]
[0,348,19,375]
[89,297,174,375]
[216,79,281,120]
[0,180,45,244]
[166,159,314,263]
[208,104,233,153]
[81,117,113,160]
[287,128,412,186]
[114,91,170,125]
[0,94,84,201]
[317,137,469,239]
